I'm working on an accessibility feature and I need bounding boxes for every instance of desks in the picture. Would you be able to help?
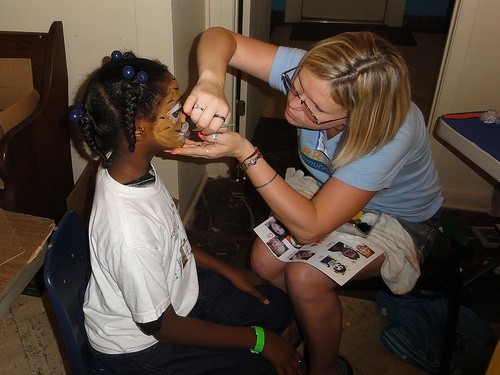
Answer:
[434,111,500,185]
[0,209,55,319]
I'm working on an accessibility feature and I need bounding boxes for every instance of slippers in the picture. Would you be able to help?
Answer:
[290,331,304,349]
[336,354,354,375]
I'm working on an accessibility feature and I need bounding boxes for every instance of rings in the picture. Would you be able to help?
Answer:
[205,147,209,156]
[213,132,220,143]
[298,357,302,362]
[213,114,225,123]
[192,104,205,112]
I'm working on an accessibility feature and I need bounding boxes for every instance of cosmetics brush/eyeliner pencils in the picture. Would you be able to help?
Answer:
[192,123,235,131]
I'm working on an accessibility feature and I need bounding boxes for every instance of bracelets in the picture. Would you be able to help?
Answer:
[250,326,265,354]
[236,147,262,181]
[255,171,278,189]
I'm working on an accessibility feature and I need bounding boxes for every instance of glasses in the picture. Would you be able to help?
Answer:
[281,65,350,125]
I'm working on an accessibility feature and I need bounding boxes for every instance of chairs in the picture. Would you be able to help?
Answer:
[335,226,462,375]
[43,209,114,373]
[0,20,77,297]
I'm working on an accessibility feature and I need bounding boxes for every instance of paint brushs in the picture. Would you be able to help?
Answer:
[192,123,235,131]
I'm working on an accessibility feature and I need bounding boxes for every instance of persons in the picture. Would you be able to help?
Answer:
[264,220,374,274]
[170,26,443,375]
[69,50,305,375]
